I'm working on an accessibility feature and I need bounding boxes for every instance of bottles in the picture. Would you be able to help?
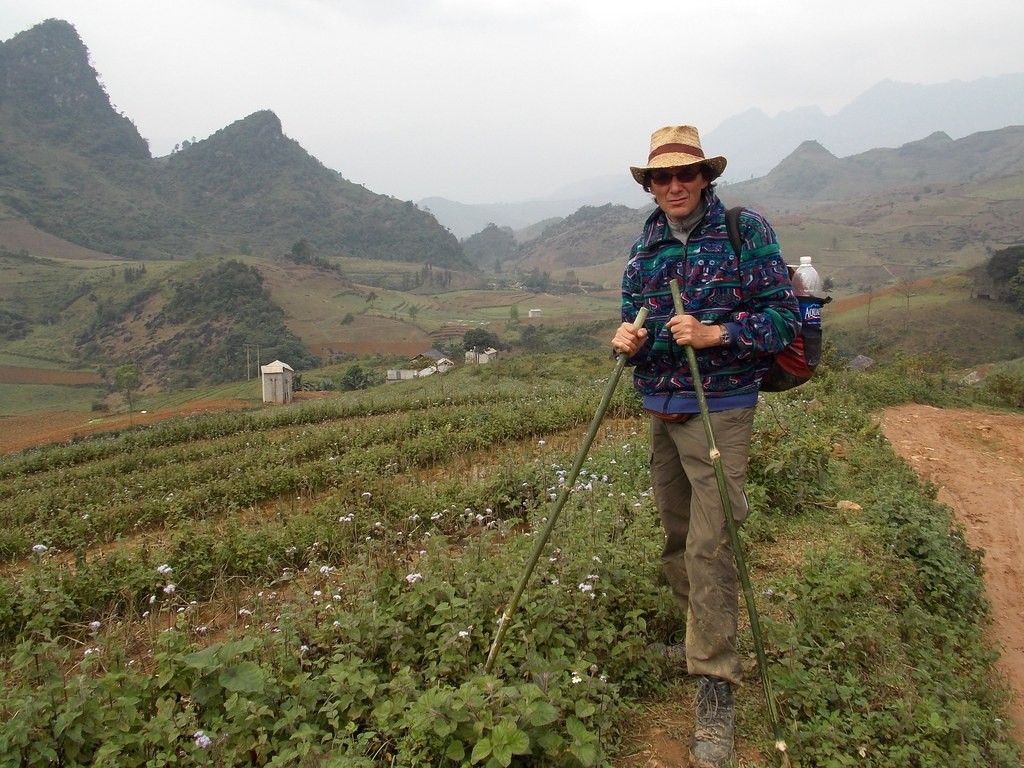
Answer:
[792,255,821,366]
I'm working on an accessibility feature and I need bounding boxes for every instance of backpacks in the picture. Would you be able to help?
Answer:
[724,205,833,393]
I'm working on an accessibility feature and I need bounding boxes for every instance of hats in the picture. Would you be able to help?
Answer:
[630,125,727,187]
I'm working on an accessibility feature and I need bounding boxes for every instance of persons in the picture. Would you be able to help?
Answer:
[605,127,796,765]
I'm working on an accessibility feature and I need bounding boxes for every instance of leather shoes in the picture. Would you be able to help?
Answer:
[689,675,735,768]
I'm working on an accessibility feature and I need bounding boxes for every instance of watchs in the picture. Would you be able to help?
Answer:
[718,324,732,346]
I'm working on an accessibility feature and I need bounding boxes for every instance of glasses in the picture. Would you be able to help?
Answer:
[650,169,702,184]
